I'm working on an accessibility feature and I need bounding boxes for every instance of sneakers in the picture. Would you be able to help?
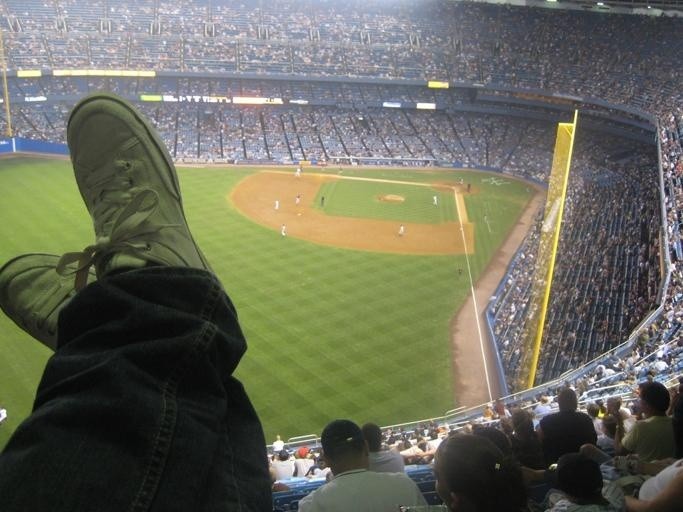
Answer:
[1,252,98,353]
[64,92,218,278]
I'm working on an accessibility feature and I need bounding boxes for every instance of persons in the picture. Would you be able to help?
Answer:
[320,196,325,206]
[467,183,471,192]
[398,224,403,236]
[262,394,488,511]
[432,194,437,207]
[3,2,682,182]
[280,223,286,237]
[489,184,683,510]
[295,196,300,205]
[275,200,279,210]
[2,89,274,510]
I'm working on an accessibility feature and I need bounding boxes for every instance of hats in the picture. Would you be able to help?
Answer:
[298,446,309,458]
[320,419,363,450]
[543,453,603,494]
